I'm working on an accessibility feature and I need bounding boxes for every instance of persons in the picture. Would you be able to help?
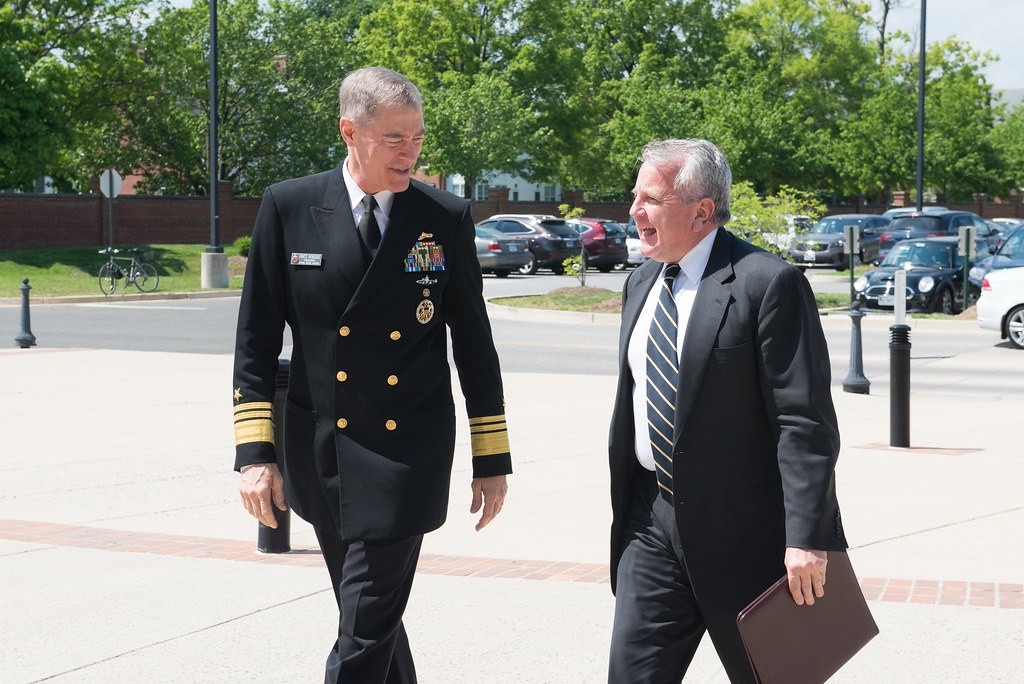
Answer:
[607,137,849,684]
[232,65,514,684]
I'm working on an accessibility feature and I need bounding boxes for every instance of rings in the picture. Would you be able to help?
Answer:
[499,505,503,508]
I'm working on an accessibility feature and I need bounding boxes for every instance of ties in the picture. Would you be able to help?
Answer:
[646,263,682,506]
[356,194,383,260]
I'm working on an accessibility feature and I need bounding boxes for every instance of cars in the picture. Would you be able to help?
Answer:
[473,224,531,279]
[968,215,1024,352]
[723,215,813,271]
[565,217,629,274]
[793,212,890,273]
[877,210,1002,262]
[883,205,949,220]
[852,234,990,315]
[612,222,654,270]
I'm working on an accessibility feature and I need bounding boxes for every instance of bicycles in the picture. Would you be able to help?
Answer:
[97,246,160,295]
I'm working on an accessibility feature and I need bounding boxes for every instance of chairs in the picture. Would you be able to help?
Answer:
[907,246,928,262]
[936,252,950,267]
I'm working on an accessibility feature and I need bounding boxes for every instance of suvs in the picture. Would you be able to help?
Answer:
[476,213,586,276]
